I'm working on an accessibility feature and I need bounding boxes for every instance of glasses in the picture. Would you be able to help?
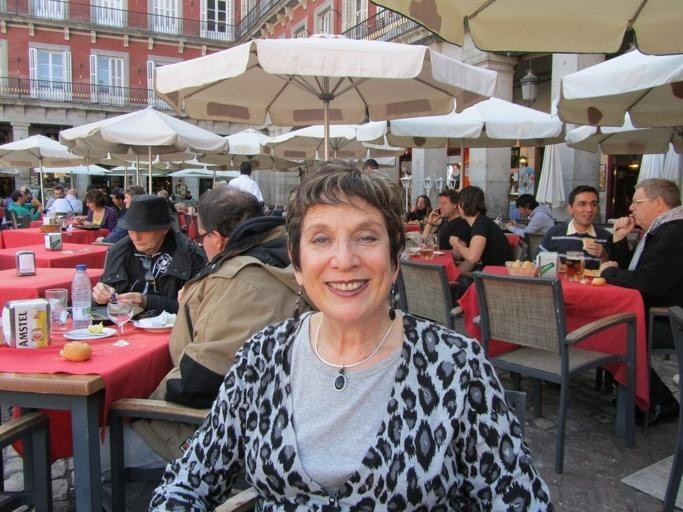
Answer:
[633,199,651,204]
[193,225,224,247]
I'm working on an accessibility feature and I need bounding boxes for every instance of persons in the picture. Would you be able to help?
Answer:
[91,194,207,316]
[97,183,314,512]
[147,157,555,512]
[533,184,614,393]
[404,185,515,319]
[0,179,194,232]
[505,194,558,239]
[598,177,683,430]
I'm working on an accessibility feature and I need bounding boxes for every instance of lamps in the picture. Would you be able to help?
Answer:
[519,155,528,167]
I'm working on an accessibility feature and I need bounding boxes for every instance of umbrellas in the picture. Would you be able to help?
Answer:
[555,48,683,130]
[153,32,498,165]
[566,112,683,156]
[354,95,566,194]
[372,1,683,57]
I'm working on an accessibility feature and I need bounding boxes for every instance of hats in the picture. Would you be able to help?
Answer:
[117,194,176,231]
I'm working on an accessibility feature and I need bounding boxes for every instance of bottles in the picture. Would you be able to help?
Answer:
[72,265,92,328]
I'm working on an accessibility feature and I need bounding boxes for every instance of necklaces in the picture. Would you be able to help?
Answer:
[314,315,397,392]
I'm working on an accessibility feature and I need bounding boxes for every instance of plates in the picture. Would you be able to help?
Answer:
[133,317,177,333]
[63,328,117,340]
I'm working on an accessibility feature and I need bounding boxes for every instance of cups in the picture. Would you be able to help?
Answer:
[108,302,135,347]
[564,251,585,281]
[420,240,433,260]
[429,234,440,252]
[45,288,68,327]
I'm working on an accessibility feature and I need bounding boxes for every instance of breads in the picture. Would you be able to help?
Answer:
[63,341,92,362]
[592,277,606,286]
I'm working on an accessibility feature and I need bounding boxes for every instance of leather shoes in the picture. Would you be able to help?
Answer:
[635,397,680,426]
